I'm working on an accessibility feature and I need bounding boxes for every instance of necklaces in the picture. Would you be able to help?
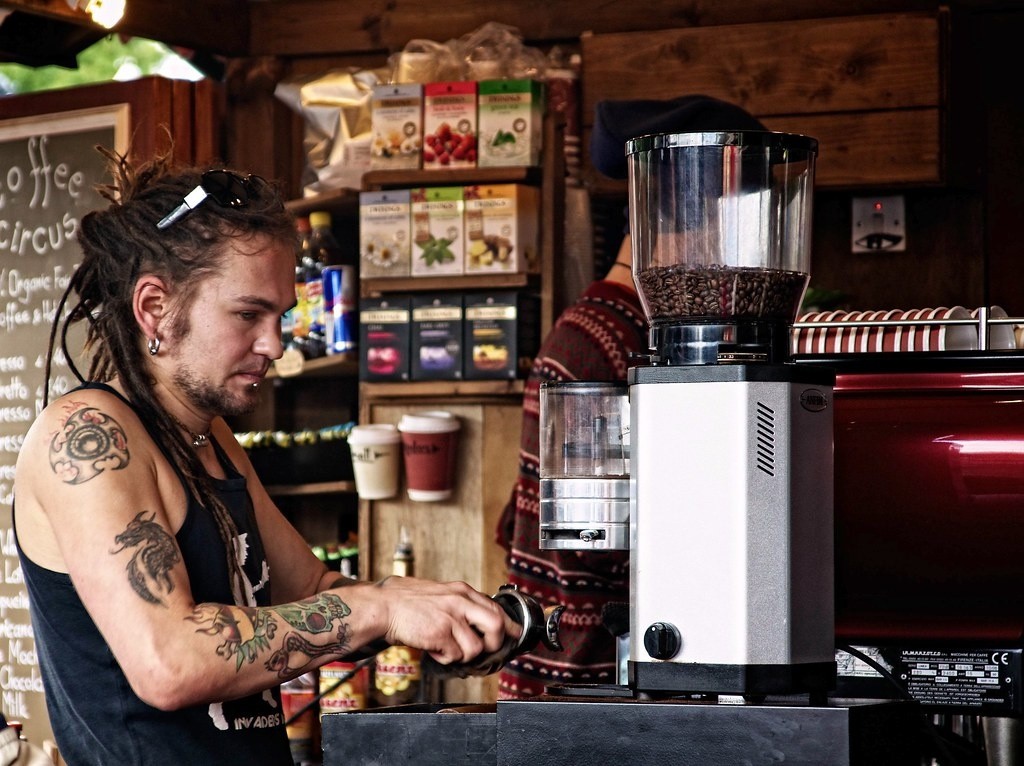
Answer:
[161,405,212,447]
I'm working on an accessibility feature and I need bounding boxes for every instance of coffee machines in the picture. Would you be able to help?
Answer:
[531,135,841,699]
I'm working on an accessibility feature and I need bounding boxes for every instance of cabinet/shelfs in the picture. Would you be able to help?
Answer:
[230,77,566,759]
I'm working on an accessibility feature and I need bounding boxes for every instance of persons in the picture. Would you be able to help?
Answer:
[14,146,522,766]
[490,94,777,705]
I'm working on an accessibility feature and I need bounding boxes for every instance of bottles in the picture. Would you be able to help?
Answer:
[280,207,339,361]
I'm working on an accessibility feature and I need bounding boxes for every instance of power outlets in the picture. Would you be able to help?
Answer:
[852,194,911,258]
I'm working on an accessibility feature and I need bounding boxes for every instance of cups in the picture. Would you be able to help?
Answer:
[346,423,403,502]
[395,409,461,503]
[782,303,1017,354]
